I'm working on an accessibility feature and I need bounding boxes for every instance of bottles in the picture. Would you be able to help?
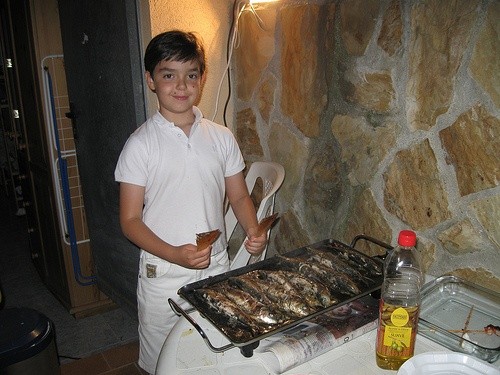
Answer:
[375,230,422,370]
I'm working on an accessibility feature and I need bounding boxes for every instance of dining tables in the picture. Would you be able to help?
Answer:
[154,270,500,375]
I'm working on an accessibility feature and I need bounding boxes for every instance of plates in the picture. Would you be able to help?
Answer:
[396,351,499,375]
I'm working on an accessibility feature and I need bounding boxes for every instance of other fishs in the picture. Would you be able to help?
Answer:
[193,244,382,333]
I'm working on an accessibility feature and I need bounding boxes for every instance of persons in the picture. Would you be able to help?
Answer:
[113,30,268,374]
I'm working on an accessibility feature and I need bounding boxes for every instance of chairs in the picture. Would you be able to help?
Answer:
[223,162,286,271]
